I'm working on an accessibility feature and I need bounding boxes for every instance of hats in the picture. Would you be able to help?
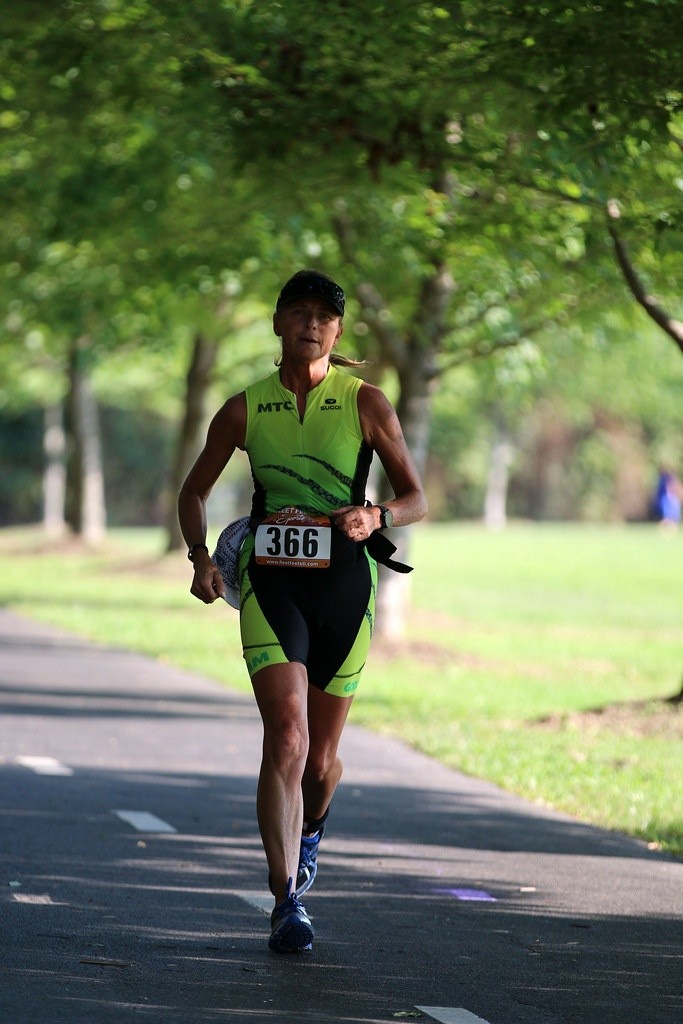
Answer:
[277,269,346,318]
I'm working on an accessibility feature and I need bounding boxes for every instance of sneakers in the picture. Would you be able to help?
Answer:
[296,805,329,899]
[267,877,315,954]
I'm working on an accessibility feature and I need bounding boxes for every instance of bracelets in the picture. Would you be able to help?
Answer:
[188,544,209,562]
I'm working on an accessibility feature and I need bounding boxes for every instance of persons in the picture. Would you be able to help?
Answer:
[654,463,683,522]
[178,269,427,953]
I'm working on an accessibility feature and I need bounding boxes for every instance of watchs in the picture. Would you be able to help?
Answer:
[374,502,393,529]
